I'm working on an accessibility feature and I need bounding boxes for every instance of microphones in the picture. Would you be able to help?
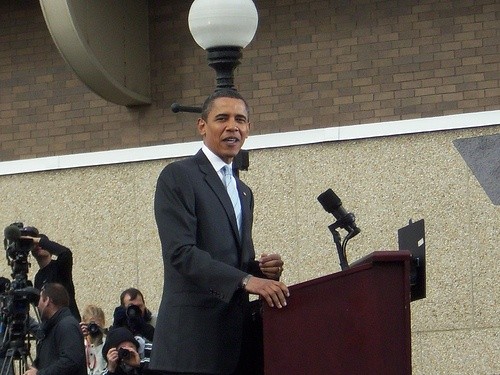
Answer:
[4,225,21,241]
[317,188,356,233]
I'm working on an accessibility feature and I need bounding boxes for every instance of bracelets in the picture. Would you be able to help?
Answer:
[241,273,253,289]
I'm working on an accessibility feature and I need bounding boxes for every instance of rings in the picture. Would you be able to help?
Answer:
[278,267,281,273]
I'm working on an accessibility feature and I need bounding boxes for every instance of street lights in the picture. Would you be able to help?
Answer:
[171,0,259,177]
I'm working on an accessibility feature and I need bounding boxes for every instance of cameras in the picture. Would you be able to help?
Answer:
[87,322,102,338]
[123,304,143,323]
[116,347,130,359]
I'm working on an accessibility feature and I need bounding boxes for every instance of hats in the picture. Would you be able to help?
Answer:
[102,326,140,363]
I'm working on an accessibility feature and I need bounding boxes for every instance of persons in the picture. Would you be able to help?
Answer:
[24,283,87,375]
[79,305,112,375]
[112,288,158,358]
[20,234,82,325]
[143,90,290,375]
[0,276,42,375]
[100,328,151,375]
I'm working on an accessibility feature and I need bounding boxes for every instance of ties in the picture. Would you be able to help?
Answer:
[222,165,242,242]
